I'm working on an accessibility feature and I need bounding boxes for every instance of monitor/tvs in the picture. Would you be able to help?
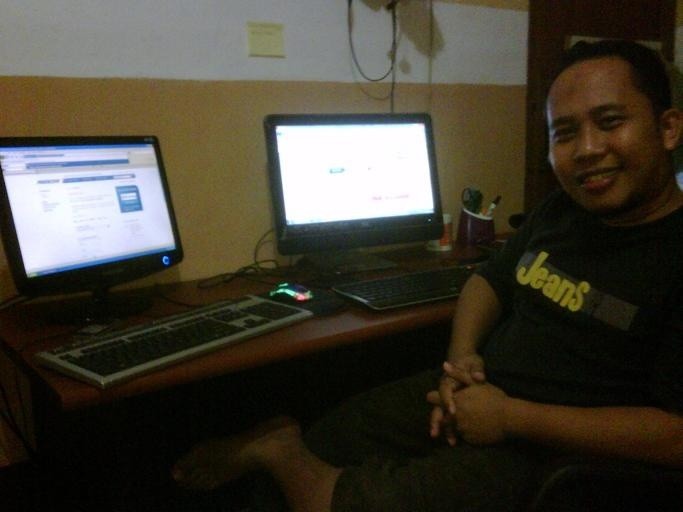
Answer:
[1,135,184,328]
[263,113,444,275]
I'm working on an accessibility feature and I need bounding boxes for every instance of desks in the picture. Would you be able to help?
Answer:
[0,235,505,512]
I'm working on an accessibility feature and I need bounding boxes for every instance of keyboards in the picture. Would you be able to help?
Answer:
[331,268,472,311]
[33,293,314,392]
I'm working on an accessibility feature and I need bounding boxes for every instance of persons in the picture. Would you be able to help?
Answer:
[169,39,682,510]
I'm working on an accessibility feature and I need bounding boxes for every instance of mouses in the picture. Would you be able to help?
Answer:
[268,282,314,303]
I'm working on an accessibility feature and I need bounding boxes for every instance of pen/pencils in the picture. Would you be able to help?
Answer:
[486,194,501,218]
